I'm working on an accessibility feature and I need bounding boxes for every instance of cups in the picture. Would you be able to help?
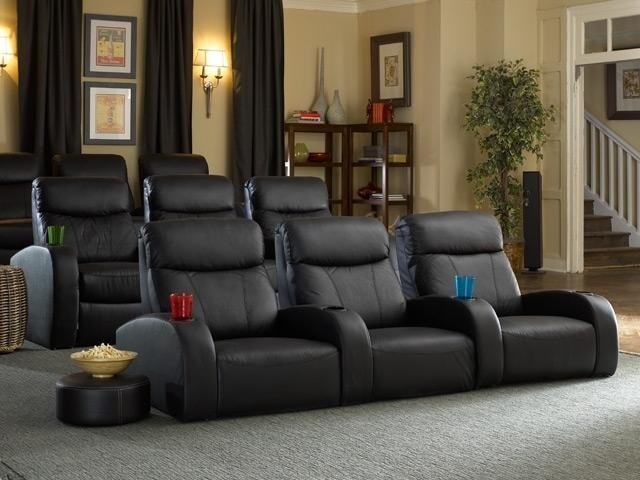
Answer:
[454,275,476,298]
[46,225,65,246]
[169,294,192,320]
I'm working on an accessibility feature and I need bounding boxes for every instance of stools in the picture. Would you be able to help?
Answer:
[57,373,151,427]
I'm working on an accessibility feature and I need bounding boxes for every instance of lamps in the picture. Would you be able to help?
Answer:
[193,48,228,118]
[0,30,12,68]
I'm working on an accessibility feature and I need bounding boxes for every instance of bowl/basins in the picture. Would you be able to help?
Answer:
[309,152,331,162]
[71,351,138,378]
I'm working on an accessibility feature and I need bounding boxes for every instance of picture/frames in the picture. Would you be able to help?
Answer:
[371,32,411,108]
[83,81,137,145]
[84,13,137,79]
[603,46,640,119]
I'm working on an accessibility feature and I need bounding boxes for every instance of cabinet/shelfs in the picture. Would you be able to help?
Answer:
[349,124,413,234]
[284,124,349,216]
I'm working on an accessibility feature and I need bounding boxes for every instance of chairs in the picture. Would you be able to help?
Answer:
[0,152,209,266]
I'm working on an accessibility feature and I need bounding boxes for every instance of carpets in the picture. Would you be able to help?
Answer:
[0,339,639,480]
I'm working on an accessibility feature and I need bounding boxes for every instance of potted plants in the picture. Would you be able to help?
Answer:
[459,58,558,282]
